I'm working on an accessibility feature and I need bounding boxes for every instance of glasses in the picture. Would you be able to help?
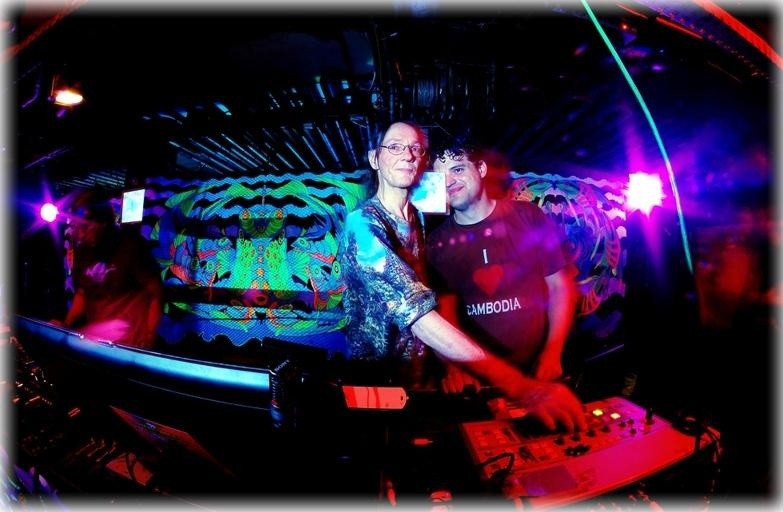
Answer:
[377,143,426,157]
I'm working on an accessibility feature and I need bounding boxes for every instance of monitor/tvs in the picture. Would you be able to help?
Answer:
[7,313,280,499]
[119,187,146,224]
[407,171,451,217]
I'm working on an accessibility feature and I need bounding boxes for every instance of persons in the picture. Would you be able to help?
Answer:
[428,138,583,397]
[43,190,163,353]
[340,118,592,435]
